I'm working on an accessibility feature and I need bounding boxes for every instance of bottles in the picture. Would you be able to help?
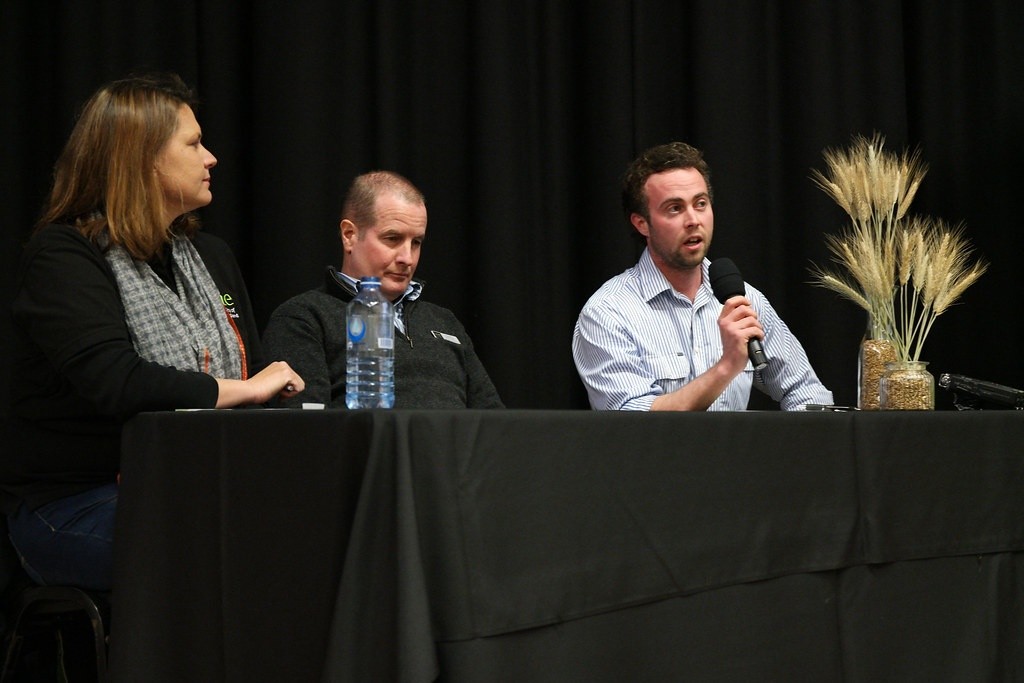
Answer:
[346,275,395,409]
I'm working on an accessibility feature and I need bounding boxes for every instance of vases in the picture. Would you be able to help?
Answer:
[878,361,934,409]
[856,322,900,410]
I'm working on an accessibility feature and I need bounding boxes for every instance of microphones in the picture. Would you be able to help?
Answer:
[707,258,769,369]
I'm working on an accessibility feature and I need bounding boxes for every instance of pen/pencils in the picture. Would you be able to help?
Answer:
[798,403,861,411]
[283,385,294,392]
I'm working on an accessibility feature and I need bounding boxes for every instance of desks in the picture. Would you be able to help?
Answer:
[106,407,1024,683]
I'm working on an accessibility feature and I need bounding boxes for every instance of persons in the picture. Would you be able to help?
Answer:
[259,170,505,410]
[571,142,835,411]
[0,78,305,589]
